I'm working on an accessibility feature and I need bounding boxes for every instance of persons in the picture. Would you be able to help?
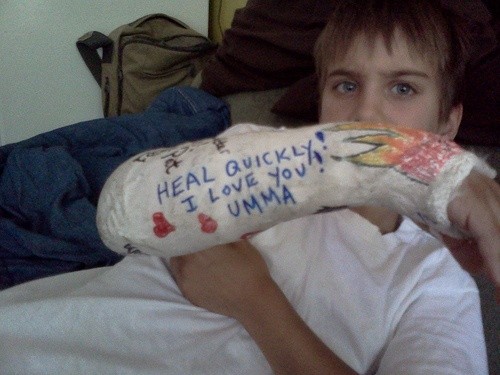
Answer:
[0,0,500,375]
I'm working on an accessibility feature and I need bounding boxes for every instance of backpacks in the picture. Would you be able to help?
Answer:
[77,13,217,118]
